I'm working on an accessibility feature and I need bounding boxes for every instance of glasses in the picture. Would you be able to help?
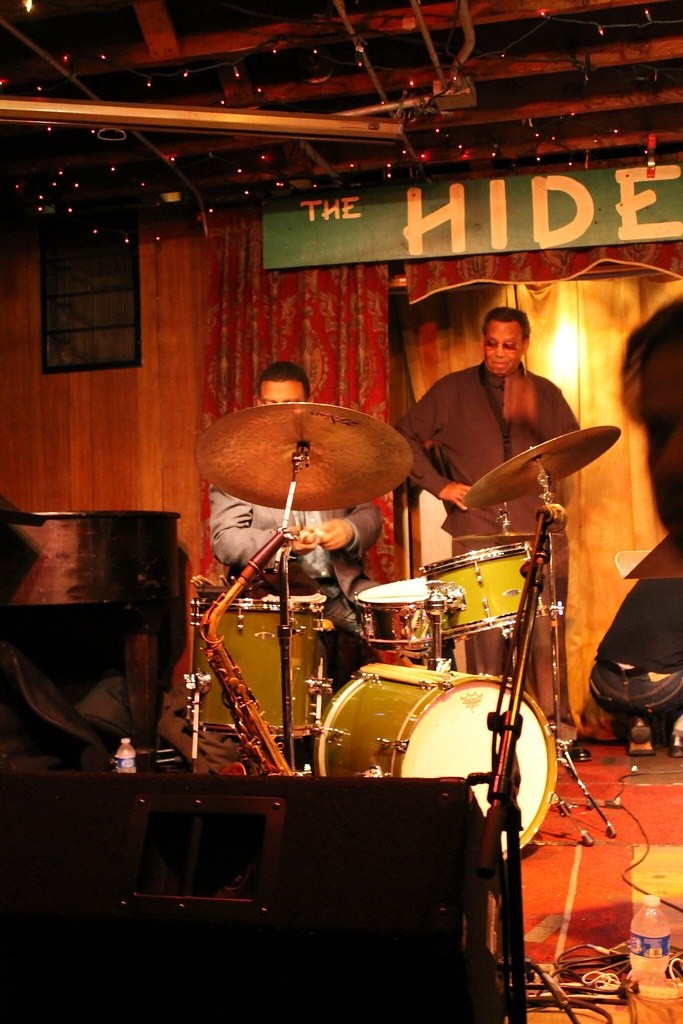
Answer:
[483,340,520,353]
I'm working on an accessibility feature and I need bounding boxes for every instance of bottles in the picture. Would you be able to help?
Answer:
[115,738,136,773]
[630,895,671,984]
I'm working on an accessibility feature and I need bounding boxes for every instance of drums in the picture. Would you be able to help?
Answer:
[356,575,441,654]
[313,663,558,860]
[420,541,548,640]
[191,598,318,738]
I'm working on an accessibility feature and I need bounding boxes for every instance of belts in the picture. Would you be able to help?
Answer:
[597,659,647,678]
[314,578,337,585]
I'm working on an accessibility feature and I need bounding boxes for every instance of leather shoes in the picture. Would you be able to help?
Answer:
[563,746,591,761]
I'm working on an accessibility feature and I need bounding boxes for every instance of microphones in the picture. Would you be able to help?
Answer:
[535,504,569,533]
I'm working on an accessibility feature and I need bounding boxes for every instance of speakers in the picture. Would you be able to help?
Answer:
[0,766,554,1024]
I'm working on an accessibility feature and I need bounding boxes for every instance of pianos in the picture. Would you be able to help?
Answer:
[0,510,182,772]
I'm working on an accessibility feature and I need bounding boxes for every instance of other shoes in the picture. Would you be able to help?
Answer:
[628,725,655,755]
[669,736,683,757]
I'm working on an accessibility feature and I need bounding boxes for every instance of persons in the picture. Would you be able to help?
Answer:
[623,297,683,546]
[589,578,683,756]
[210,360,381,632]
[393,307,592,760]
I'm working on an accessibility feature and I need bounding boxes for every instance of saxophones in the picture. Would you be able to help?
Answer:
[197,525,317,777]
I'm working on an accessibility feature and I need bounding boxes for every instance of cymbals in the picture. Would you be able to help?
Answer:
[461,425,622,509]
[193,401,415,512]
